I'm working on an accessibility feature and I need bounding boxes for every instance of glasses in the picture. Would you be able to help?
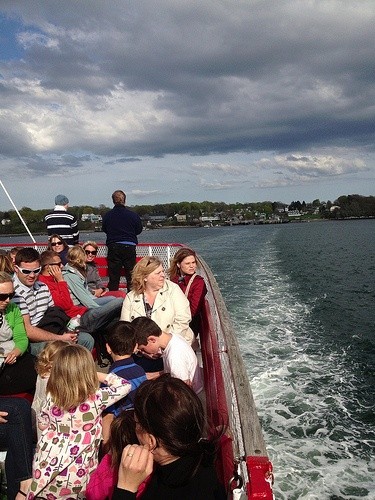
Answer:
[85,250,98,255]
[146,256,159,267]
[127,408,158,444]
[0,292,15,301]
[51,241,63,246]
[16,265,41,276]
[44,261,64,268]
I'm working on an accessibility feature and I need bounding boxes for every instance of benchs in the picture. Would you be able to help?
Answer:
[93,256,142,288]
[198,297,236,500]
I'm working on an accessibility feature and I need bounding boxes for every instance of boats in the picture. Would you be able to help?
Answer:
[0,242,277,500]
[93,226,102,232]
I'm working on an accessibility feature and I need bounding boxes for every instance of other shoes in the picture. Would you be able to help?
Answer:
[100,353,111,368]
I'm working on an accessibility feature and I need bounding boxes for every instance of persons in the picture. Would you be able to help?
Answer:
[102,190,143,294]
[44,194,79,252]
[0,234,229,500]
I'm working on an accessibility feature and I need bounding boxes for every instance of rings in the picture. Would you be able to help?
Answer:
[127,454,133,457]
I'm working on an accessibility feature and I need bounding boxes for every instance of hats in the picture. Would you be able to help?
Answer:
[55,195,69,205]
